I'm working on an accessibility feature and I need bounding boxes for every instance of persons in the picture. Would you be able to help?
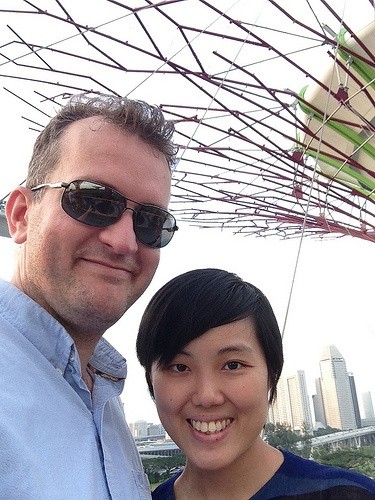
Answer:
[136,268,375,500]
[0,94,179,500]
[78,205,93,222]
[69,189,78,211]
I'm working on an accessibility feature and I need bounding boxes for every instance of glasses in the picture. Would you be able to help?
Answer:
[30,180,179,249]
